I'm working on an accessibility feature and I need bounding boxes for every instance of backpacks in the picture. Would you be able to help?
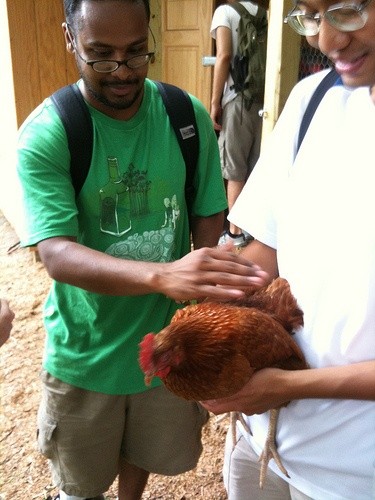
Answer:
[226,0,268,112]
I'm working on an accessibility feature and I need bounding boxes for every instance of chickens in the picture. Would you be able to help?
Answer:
[138,277,312,488]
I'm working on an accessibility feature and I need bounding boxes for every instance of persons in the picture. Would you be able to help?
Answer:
[207,0,269,250]
[198,0,375,500]
[16,0,272,500]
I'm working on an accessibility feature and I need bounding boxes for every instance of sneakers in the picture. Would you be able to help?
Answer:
[217,228,254,248]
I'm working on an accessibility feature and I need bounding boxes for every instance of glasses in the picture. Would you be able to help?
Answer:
[284,0,368,36]
[65,22,156,74]
